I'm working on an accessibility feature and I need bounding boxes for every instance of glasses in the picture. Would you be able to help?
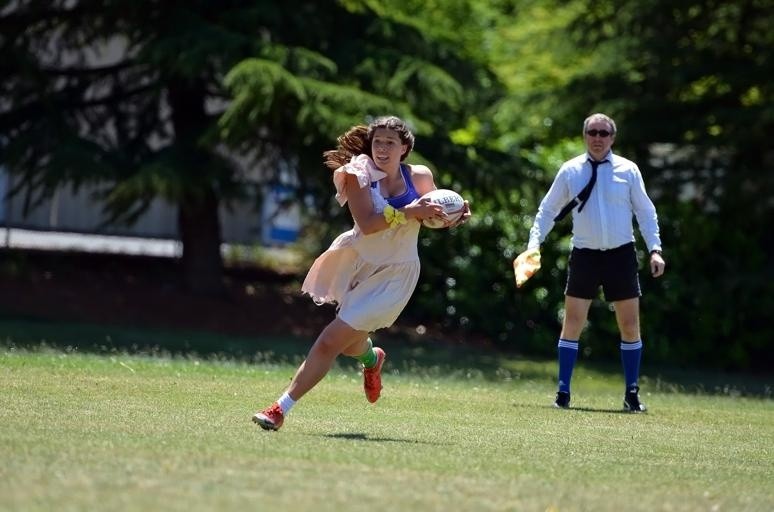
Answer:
[584,129,613,137]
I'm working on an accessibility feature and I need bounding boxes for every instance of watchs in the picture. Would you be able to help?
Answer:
[649,249,663,257]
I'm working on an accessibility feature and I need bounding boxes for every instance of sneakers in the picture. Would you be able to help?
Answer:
[555,390,571,409]
[252,402,284,431]
[362,346,387,404]
[622,386,646,413]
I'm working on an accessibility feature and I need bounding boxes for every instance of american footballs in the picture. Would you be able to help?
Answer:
[416,189,465,229]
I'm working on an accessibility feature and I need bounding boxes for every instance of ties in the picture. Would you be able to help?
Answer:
[554,156,612,222]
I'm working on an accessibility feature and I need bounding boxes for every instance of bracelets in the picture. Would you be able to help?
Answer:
[382,204,407,231]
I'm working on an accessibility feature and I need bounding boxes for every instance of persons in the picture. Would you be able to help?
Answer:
[512,112,664,414]
[250,114,472,430]
